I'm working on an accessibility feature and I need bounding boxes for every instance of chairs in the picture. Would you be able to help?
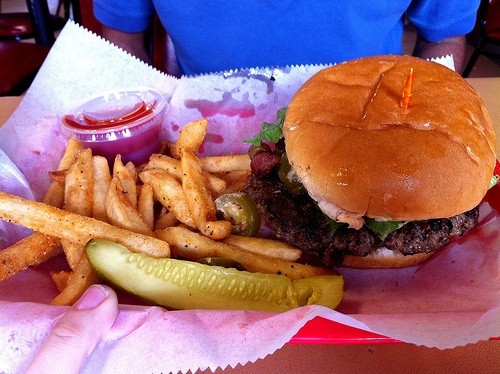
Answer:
[462,0,500,76]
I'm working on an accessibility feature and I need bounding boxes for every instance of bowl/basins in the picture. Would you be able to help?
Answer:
[58,87,169,170]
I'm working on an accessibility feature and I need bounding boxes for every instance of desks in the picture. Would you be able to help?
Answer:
[0,79,500,373]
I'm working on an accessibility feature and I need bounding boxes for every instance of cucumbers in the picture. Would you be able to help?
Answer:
[85,239,344,312]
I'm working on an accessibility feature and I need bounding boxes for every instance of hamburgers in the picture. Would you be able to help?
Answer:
[242,55,500,269]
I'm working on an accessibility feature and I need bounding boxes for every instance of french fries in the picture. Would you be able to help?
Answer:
[0,119,342,305]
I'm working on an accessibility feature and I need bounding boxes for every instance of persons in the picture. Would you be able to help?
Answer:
[92,0,483,78]
